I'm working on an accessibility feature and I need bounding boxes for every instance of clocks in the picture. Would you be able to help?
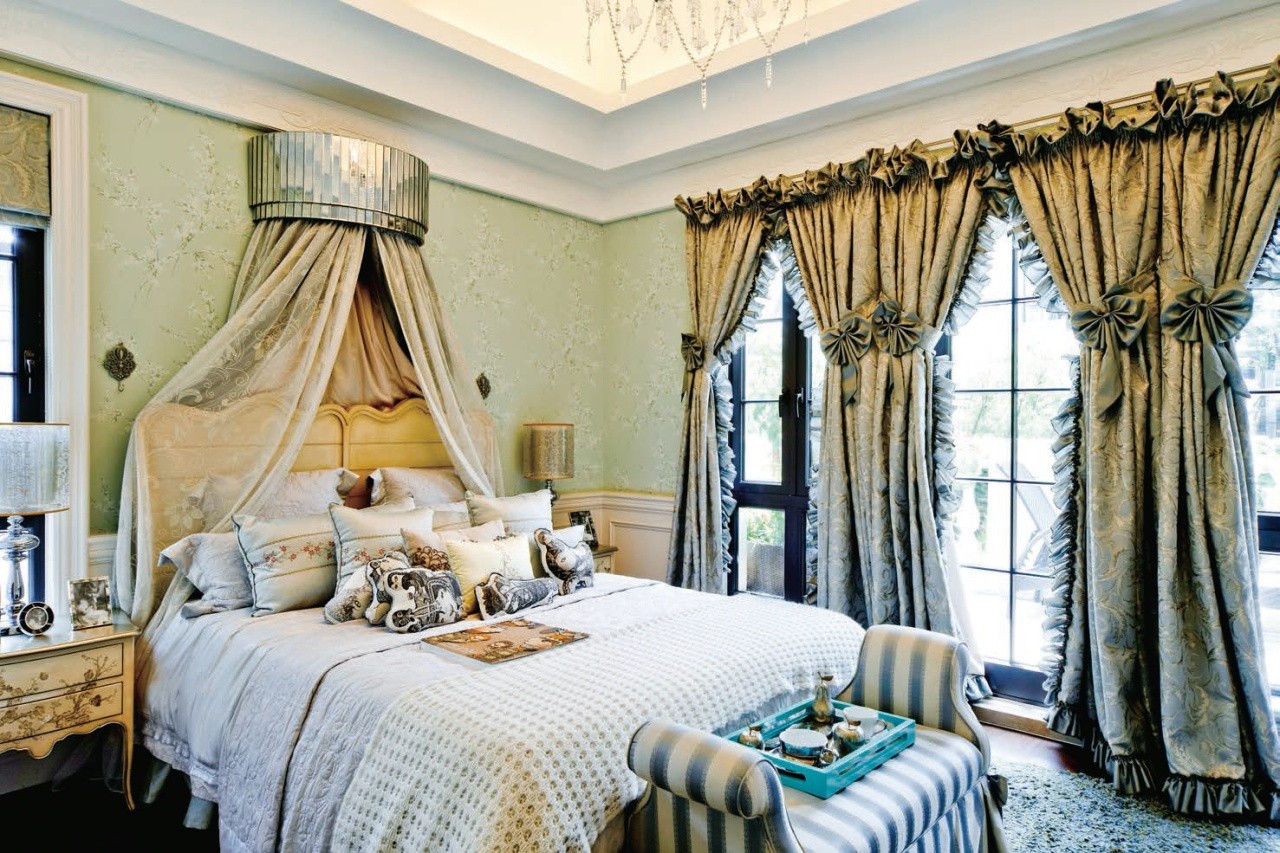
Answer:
[17,602,55,636]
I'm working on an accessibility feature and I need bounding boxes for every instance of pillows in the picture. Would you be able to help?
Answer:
[447,531,537,615]
[323,563,374,624]
[400,517,506,571]
[157,531,253,620]
[365,551,413,625]
[535,528,594,595]
[365,466,492,507]
[229,489,418,618]
[327,501,434,598]
[426,499,472,534]
[383,568,465,634]
[181,467,360,533]
[466,489,554,579]
[474,571,560,621]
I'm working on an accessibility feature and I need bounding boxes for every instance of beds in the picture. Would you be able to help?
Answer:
[132,398,868,853]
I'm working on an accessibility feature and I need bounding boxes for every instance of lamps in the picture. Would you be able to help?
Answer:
[585,1,819,111]
[0,420,70,637]
[522,423,575,528]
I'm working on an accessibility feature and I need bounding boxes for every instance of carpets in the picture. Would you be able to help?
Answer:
[992,759,1280,853]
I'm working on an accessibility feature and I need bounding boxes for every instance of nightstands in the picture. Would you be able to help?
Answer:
[590,542,619,574]
[0,600,143,811]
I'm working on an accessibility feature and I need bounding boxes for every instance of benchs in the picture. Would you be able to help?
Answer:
[625,623,1009,853]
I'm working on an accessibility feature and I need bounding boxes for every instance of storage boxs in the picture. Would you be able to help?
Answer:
[720,695,916,799]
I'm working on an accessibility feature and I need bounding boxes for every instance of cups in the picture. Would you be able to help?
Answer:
[844,706,887,734]
[780,730,840,768]
